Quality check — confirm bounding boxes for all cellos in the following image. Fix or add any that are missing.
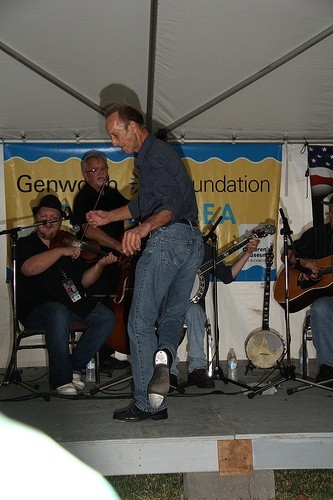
[106,260,133,356]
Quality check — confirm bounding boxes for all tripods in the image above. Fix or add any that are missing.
[167,207,333,400]
[0,214,74,401]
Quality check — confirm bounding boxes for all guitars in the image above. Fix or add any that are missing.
[244,246,285,369]
[273,255,333,313]
[189,225,276,303]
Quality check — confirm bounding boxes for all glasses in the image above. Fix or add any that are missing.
[86,167,108,172]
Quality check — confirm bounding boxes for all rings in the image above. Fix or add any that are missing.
[121,249,127,253]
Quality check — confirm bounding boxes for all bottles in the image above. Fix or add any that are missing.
[228,348,238,383]
[85,358,96,383]
[300,345,309,375]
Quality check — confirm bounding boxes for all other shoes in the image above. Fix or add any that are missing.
[187,368,215,388]
[101,356,129,370]
[56,374,85,396]
[113,405,168,423]
[147,348,174,409]
[316,364,333,388]
[168,373,178,393]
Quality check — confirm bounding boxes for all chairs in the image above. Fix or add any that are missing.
[302,311,313,380]
[3,247,101,385]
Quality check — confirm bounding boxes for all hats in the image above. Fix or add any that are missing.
[32,194,67,217]
[321,195,333,205]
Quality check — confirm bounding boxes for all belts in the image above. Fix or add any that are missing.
[177,219,198,227]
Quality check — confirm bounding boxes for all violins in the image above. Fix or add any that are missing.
[50,230,133,269]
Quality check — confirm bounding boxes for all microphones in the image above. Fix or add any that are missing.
[67,206,80,233]
[290,263,312,275]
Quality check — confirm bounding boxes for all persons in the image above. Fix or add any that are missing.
[102,103,204,422]
[169,234,260,393]
[70,150,149,370]
[13,195,117,395]
[281,196,333,382]
